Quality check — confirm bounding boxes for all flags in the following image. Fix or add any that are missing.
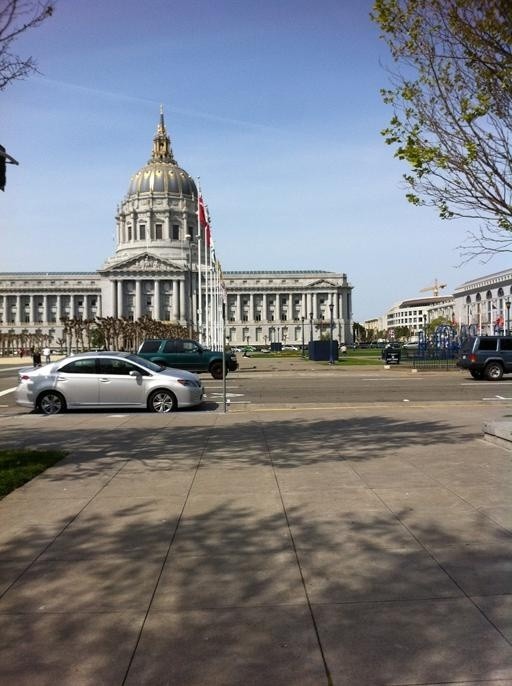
[198,196,226,303]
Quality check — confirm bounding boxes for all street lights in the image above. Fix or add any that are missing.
[301,303,335,355]
[504,302,511,335]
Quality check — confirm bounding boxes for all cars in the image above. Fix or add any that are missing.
[404,342,425,351]
[15,350,208,414]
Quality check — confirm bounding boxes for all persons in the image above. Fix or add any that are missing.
[43,346,52,365]
[20,345,26,357]
[31,345,36,358]
[33,349,42,367]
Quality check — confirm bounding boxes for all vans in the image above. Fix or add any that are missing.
[359,342,398,348]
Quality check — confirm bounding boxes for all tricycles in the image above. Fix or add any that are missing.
[381,348,401,364]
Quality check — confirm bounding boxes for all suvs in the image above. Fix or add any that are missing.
[138,338,239,379]
[456,334,511,380]
